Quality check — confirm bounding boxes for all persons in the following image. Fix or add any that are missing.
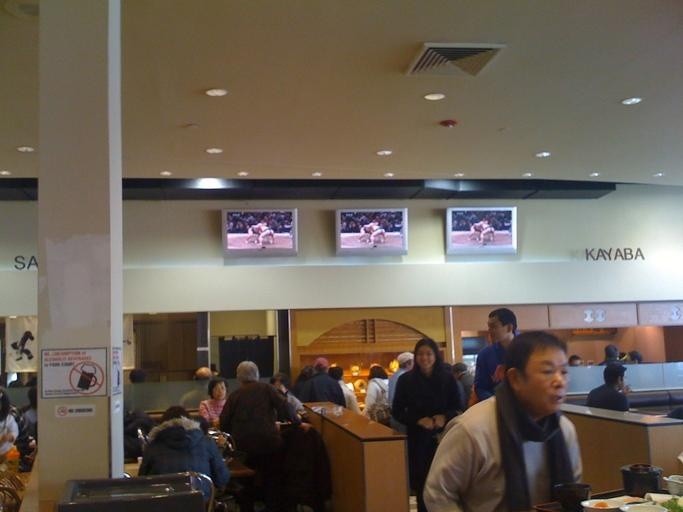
[388,352,416,496]
[452,211,512,245]
[444,363,480,411]
[124,399,157,463]
[392,339,460,512]
[598,345,640,366]
[2,372,37,472]
[423,331,584,512]
[196,361,315,512]
[475,308,517,400]
[227,212,292,249]
[342,212,402,248]
[139,405,230,512]
[304,357,360,413]
[585,363,630,411]
[361,363,393,429]
[129,369,145,383]
[569,355,582,367]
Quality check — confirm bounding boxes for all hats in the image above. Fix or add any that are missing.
[312,358,331,371]
[397,352,414,366]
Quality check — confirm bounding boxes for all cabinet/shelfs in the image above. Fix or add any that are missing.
[343,372,393,409]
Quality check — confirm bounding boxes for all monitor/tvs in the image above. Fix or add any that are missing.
[462,348,481,377]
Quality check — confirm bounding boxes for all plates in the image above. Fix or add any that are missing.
[579,492,667,512]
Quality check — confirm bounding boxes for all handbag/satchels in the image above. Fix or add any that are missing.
[368,379,391,424]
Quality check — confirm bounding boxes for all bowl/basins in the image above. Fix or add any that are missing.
[552,482,590,508]
[663,473,683,495]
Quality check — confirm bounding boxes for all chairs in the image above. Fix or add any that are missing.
[137,428,149,449]
